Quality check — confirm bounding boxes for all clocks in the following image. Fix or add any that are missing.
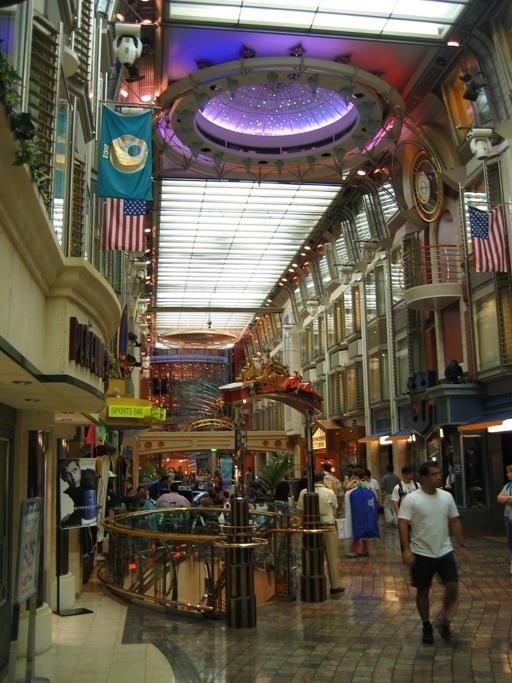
[410,151,443,223]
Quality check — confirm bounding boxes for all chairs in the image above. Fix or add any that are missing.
[114,495,232,548]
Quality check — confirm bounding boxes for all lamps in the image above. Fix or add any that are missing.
[149,44,407,185]
[160,330,237,351]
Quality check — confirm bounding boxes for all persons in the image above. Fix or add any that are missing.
[296,472,347,593]
[319,461,418,560]
[397,460,465,643]
[497,465,512,574]
[60,459,96,527]
[117,466,274,509]
[443,462,455,488]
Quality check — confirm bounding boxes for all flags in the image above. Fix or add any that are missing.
[101,196,146,253]
[95,100,156,200]
[468,202,509,273]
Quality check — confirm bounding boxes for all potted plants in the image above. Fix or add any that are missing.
[250,448,298,560]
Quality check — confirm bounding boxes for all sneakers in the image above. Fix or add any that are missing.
[421,620,454,645]
[328,586,347,598]
[343,549,370,559]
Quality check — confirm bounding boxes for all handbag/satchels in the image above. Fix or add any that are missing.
[335,518,346,541]
